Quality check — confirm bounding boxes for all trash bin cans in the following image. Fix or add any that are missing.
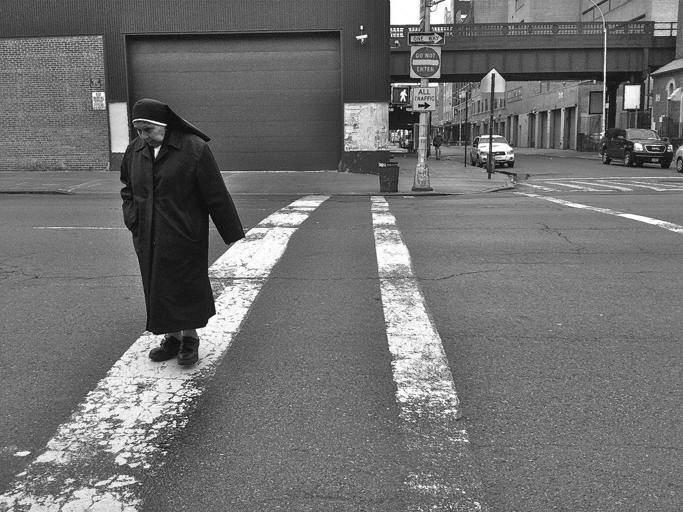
[377,160,399,192]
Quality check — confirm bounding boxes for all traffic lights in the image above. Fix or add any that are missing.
[392,86,410,105]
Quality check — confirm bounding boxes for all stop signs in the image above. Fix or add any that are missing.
[411,47,440,79]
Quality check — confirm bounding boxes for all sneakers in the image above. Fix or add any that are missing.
[149,335,181,361]
[177,335,200,366]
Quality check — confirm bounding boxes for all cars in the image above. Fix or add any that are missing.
[676,144,682,173]
[390,132,411,147]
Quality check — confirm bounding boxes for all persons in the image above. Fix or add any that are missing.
[118,97,246,368]
[432,131,444,160]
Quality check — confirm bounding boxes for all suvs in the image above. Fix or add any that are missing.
[598,128,673,170]
[470,133,515,169]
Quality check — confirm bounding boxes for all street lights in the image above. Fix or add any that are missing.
[448,95,462,148]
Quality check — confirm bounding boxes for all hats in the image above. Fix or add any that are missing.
[132,98,169,126]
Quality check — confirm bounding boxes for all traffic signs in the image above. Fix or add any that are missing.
[408,32,444,46]
[411,87,437,113]
